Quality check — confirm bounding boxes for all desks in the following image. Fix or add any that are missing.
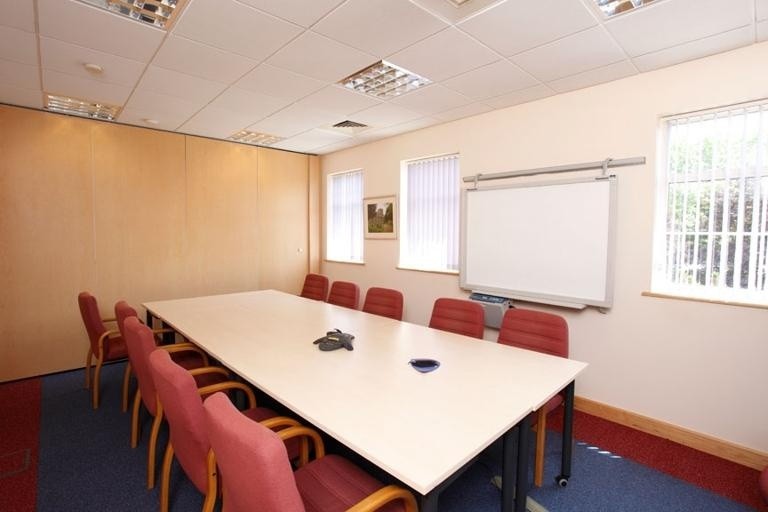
[139,290,593,512]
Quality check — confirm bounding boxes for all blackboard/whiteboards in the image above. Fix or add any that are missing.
[460,172,620,310]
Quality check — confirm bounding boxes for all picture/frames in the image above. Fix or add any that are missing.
[362,194,400,241]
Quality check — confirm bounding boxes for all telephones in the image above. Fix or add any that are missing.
[313,328,355,350]
